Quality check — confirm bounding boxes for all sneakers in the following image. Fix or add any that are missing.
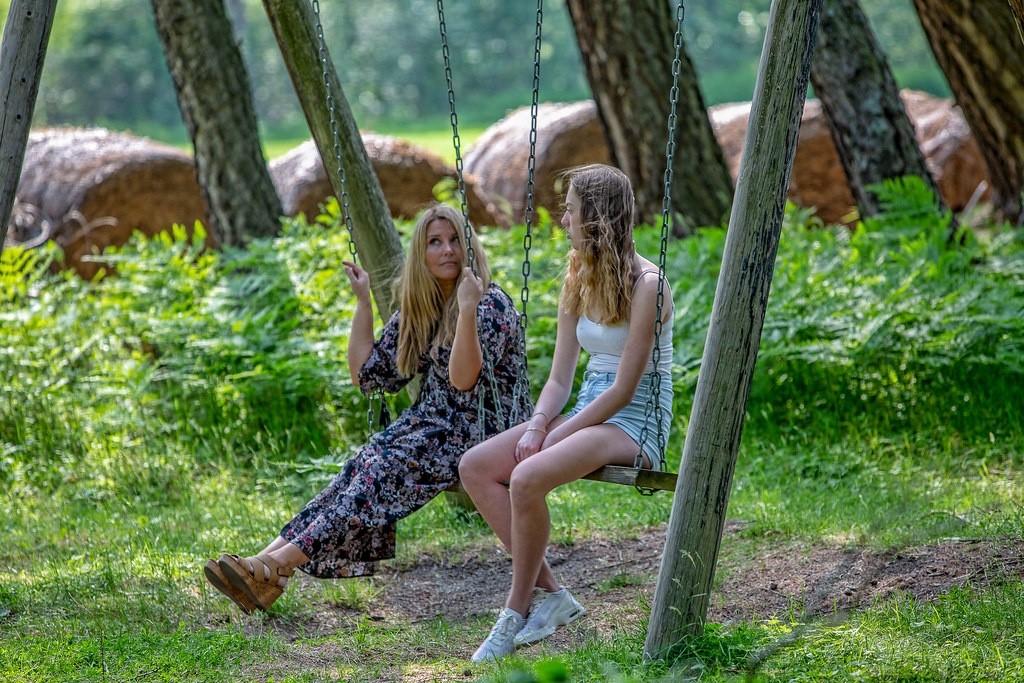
[470,607,528,666]
[513,585,586,651]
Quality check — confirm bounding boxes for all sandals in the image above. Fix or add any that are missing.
[218,554,295,612]
[204,559,256,616]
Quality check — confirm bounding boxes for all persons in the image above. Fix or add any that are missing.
[458,162,675,664]
[204,206,533,621]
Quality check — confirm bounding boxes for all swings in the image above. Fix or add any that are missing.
[506,0,688,496]
[310,0,477,494]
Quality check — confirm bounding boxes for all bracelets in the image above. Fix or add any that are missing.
[526,411,550,434]
